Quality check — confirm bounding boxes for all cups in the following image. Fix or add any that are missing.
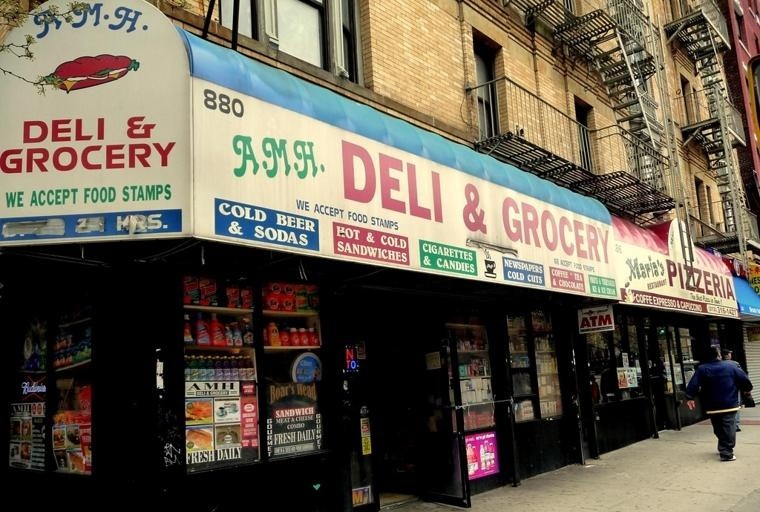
[231,404,236,412]
[219,407,225,416]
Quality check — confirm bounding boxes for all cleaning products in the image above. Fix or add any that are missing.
[242,317,255,347]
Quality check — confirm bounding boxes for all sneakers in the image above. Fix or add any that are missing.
[736,429,741,431]
[721,456,736,460]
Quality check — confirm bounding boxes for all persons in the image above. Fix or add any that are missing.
[685,344,755,461]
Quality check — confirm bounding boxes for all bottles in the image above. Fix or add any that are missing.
[184,355,255,382]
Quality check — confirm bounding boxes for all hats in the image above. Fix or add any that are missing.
[722,348,734,353]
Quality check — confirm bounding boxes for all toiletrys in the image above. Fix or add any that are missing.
[194,312,213,346]
[229,321,245,347]
[224,322,235,347]
[208,312,228,346]
[183,314,198,344]
